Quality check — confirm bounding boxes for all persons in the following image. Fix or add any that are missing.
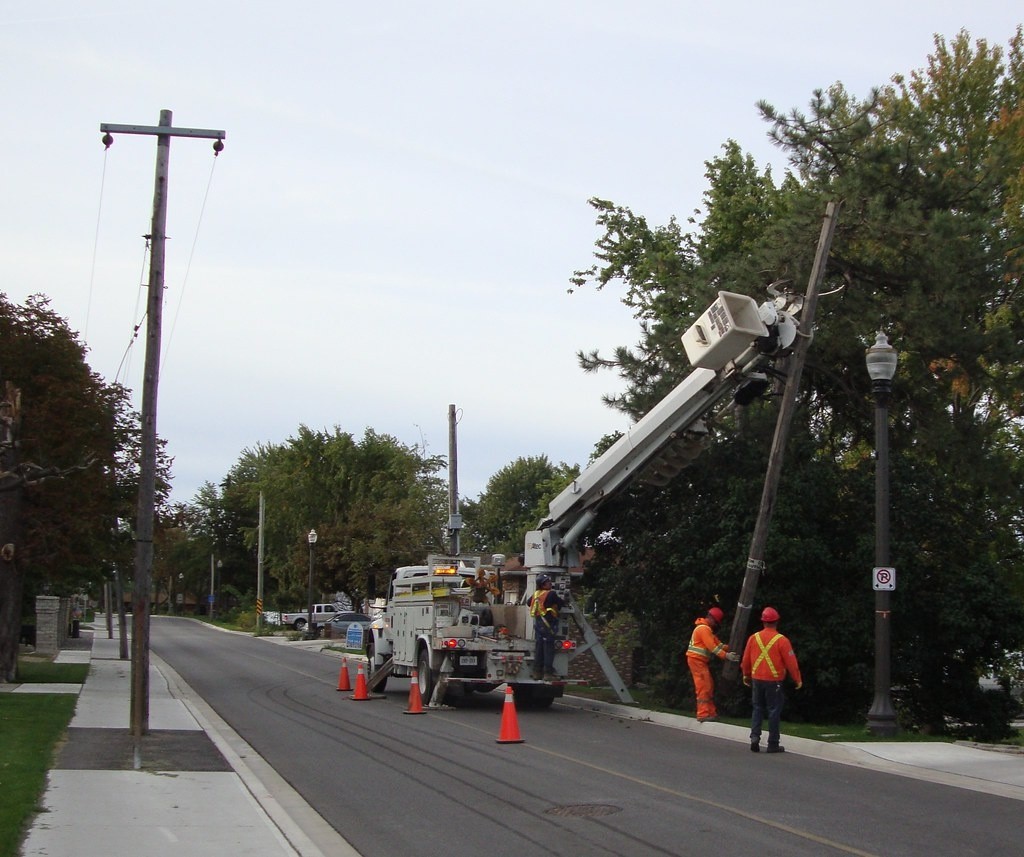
[686,608,741,722]
[742,608,802,753]
[527,573,562,681]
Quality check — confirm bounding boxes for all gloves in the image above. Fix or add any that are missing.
[743,676,752,689]
[795,682,803,690]
[725,652,741,662]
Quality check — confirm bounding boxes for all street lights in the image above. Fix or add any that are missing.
[864,333,899,735]
[178,573,184,616]
[217,560,223,615]
[308,529,317,630]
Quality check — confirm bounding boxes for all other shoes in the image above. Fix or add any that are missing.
[697,716,719,722]
[532,672,545,680]
[750,743,760,752]
[767,746,784,753]
[543,674,562,681]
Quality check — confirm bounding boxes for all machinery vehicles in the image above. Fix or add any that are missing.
[365,278,845,709]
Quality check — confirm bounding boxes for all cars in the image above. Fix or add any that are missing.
[303,613,374,632]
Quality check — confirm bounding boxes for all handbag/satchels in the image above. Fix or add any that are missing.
[775,677,795,699]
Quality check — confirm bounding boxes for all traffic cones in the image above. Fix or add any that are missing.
[402,671,428,715]
[349,664,372,701]
[335,657,354,691]
[496,686,527,744]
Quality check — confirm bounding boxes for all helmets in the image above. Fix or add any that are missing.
[535,574,549,590]
[709,607,724,624]
[760,606,781,622]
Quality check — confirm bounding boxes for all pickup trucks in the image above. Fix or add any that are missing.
[277,604,344,630]
